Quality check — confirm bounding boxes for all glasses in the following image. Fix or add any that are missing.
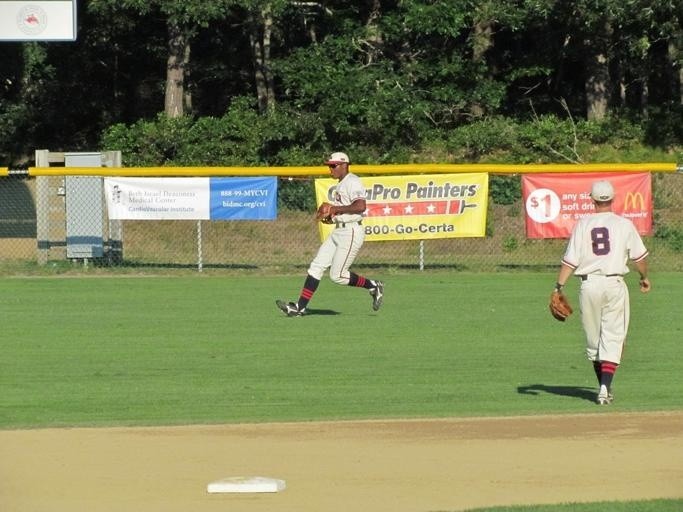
[329,164,338,168]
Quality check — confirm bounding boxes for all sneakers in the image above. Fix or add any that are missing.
[369,280,384,310]
[598,391,614,406]
[276,300,306,317]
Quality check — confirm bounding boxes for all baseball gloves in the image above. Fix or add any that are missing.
[315,203,335,223]
[551,289,573,322]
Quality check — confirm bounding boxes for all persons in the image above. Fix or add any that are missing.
[275,151,383,317]
[548,180,651,404]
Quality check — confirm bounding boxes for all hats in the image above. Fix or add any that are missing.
[324,152,349,164]
[591,181,614,202]
